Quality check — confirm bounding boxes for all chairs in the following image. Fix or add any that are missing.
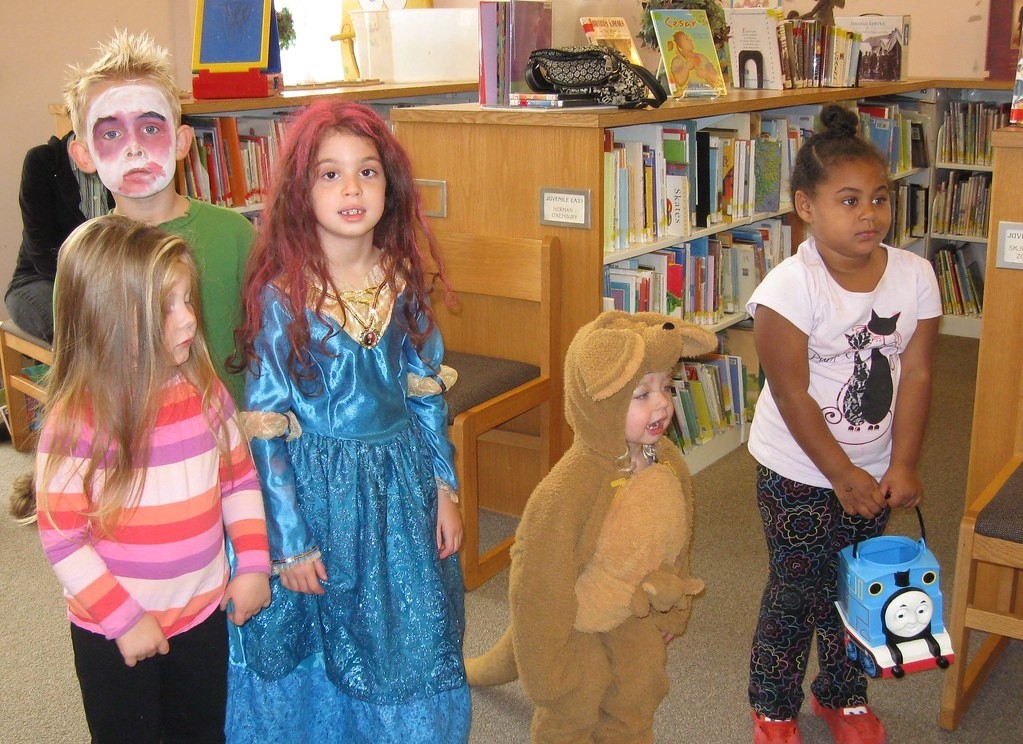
[0,319,53,452]
[409,229,562,590]
[939,402,1023,733]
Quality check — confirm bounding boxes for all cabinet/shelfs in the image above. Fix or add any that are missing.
[387,80,1023,618]
[48,80,481,226]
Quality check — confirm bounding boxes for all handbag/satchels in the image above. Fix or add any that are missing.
[525,47,669,110]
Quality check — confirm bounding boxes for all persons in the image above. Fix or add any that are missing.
[466,309,720,744]
[35,213,274,743]
[231,102,473,743]
[4,26,265,526]
[746,104,942,744]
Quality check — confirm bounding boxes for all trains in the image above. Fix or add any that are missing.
[834,535,956,679]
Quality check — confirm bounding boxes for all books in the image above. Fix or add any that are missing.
[179,93,464,237]
[510,8,1023,462]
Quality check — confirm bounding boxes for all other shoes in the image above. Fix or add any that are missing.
[753,706,800,743]
[811,689,885,744]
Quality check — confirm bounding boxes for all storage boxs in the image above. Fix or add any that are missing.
[835,13,912,82]
